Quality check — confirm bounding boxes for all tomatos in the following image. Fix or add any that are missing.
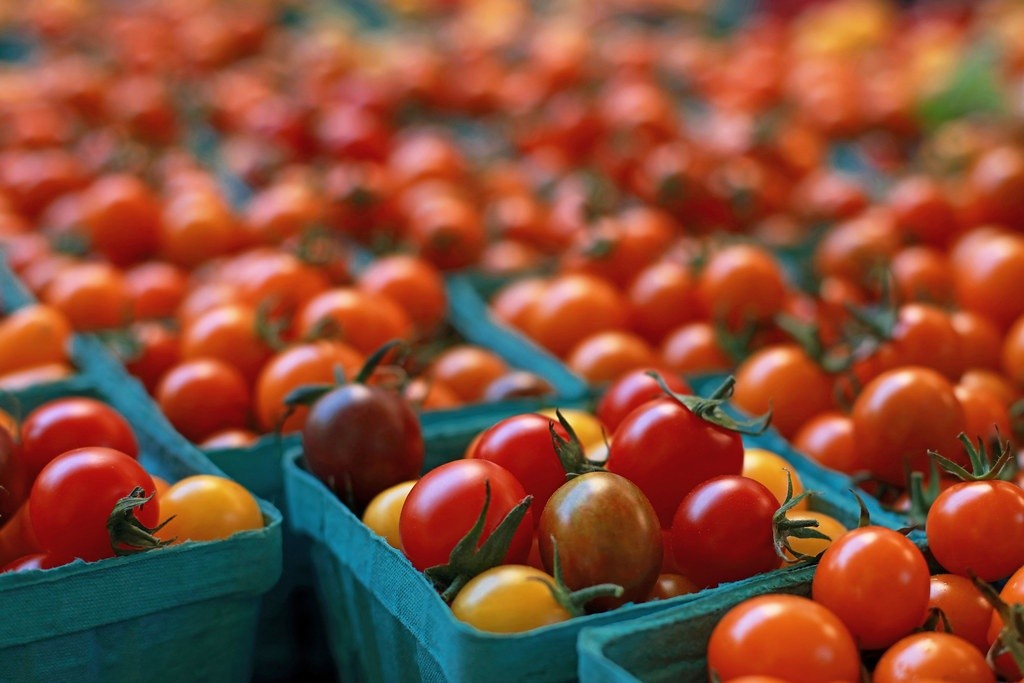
[0,0,1024,683]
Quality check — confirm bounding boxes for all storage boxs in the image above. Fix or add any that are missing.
[0,0,1024,683]
